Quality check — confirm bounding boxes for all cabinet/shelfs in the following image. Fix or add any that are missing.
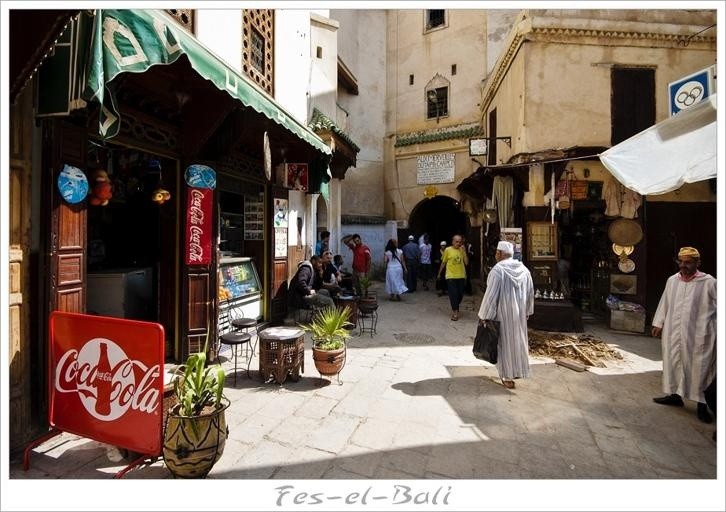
[217,253,265,340]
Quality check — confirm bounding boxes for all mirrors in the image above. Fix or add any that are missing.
[524,219,559,263]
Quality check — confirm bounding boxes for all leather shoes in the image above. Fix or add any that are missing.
[653,396,683,407]
[697,405,712,423]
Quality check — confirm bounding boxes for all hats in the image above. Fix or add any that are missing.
[678,247,701,258]
[408,235,415,241]
[496,241,514,251]
[440,241,447,246]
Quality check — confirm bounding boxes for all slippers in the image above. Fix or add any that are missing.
[451,314,458,321]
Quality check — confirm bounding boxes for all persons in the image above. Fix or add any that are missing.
[344,233,371,298]
[478,240,535,390]
[650,246,717,424]
[290,230,355,314]
[383,231,475,321]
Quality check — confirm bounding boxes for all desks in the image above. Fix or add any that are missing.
[528,297,586,333]
[258,325,306,385]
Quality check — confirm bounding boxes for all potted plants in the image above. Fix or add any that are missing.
[299,303,355,379]
[163,336,234,478]
[350,270,378,315]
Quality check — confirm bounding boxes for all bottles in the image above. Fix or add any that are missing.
[93,342,111,416]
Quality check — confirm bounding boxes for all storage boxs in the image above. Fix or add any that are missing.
[606,307,647,335]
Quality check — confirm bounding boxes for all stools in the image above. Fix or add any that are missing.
[215,330,256,389]
[231,316,259,359]
[292,300,316,325]
